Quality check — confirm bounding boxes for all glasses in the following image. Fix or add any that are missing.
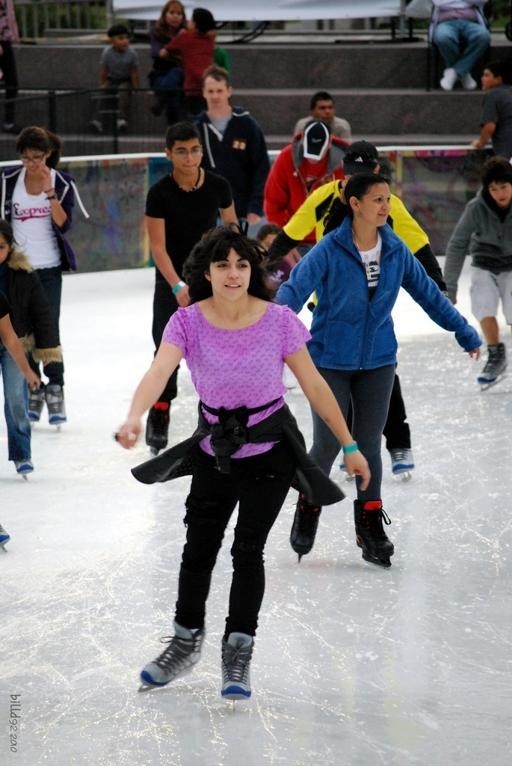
[21,151,46,163]
[173,147,202,156]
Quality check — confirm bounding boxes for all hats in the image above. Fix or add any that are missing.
[304,120,329,161]
[343,141,380,176]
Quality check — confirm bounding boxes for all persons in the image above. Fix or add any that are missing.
[0,0,512,703]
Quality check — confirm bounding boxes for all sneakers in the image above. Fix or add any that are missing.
[45,384,66,424]
[441,67,458,92]
[89,121,103,132]
[3,124,21,135]
[291,493,322,554]
[340,462,346,472]
[390,449,414,474]
[14,462,33,473]
[147,404,169,448]
[354,499,393,558]
[459,73,478,90]
[222,634,254,700]
[27,383,44,422]
[117,120,128,132]
[0,526,10,546]
[478,345,505,383]
[141,620,203,687]
